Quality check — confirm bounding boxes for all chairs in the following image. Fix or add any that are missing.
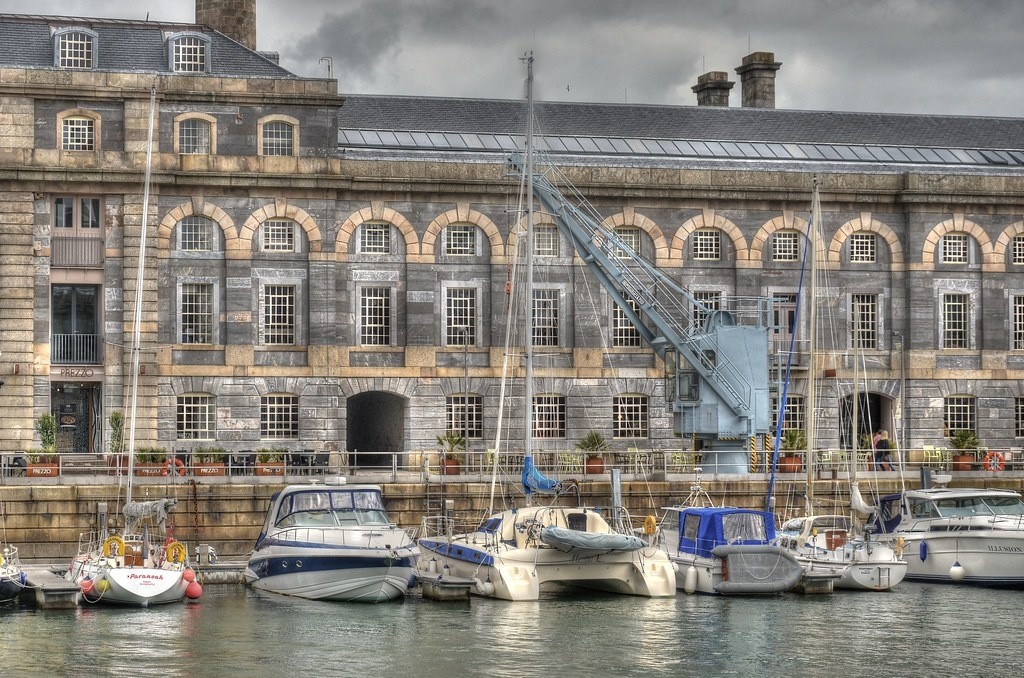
[614,447,697,474]
[175,448,190,472]
[222,450,257,476]
[1012,451,1024,472]
[0,452,27,477]
[286,448,330,476]
[473,448,583,474]
[923,445,950,468]
[819,451,869,471]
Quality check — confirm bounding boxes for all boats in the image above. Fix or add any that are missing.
[242,473,422,603]
[1,544,27,597]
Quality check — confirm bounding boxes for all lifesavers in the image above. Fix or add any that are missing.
[644,516,656,535]
[20,572,26,585]
[161,458,185,477]
[103,536,125,557]
[983,452,1005,471]
[166,542,185,562]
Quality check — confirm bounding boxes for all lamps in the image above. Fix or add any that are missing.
[14,364,19,375]
[825,369,836,378]
[140,365,145,374]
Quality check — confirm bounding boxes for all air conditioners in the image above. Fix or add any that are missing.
[970,446,989,465]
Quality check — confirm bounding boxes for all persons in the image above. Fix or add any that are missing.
[871,428,896,471]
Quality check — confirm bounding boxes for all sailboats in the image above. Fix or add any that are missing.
[420,52,1024,606]
[68,84,201,609]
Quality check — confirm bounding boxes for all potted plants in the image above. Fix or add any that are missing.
[134,447,168,476]
[192,446,226,477]
[573,431,612,474]
[106,411,129,475]
[436,431,467,475]
[25,413,59,477]
[779,429,807,473]
[949,430,979,470]
[256,448,286,476]
